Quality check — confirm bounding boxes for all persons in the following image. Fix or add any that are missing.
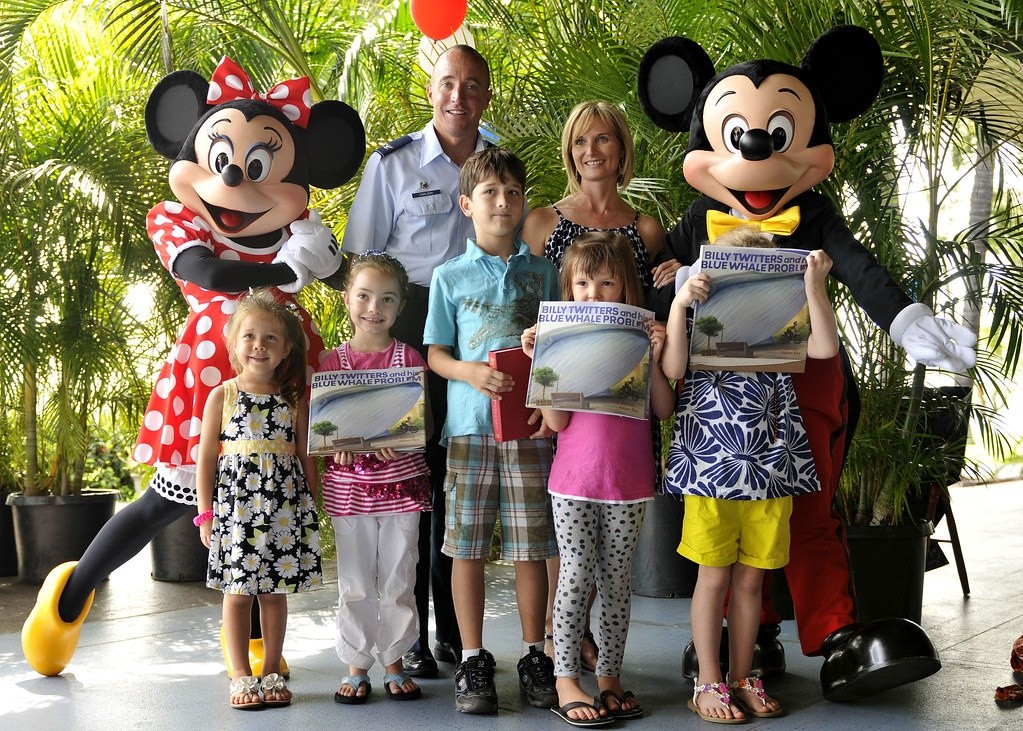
[197,284,317,709]
[341,45,502,679]
[520,99,683,676]
[520,230,676,727]
[660,229,840,723]
[422,147,563,713]
[320,251,430,705]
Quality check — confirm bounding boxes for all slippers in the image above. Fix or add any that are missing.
[230,675,262,710]
[383,671,421,700]
[261,672,291,705]
[599,685,644,717]
[335,674,371,704]
[551,692,615,726]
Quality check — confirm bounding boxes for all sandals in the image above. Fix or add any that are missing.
[686,673,748,723]
[725,671,784,716]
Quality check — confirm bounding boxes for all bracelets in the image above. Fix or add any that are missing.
[193,510,213,527]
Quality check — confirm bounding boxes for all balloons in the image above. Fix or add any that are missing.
[410,0,467,41]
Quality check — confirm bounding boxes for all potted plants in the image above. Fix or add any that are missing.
[0,0,1023,623]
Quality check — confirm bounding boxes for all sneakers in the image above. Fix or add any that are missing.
[455,649,499,715]
[517,644,560,708]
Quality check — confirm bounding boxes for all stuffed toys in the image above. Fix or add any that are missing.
[20,54,365,676]
[635,23,979,701]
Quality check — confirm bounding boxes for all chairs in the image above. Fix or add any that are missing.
[871,387,969,596]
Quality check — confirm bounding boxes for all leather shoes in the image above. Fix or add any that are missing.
[403,650,439,674]
[435,640,495,668]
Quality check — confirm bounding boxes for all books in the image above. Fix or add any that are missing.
[488,346,543,443]
[690,245,815,374]
[524,300,656,421]
[306,366,427,456]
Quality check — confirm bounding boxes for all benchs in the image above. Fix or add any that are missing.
[715,341,753,358]
[332,436,370,451]
[551,393,590,409]
